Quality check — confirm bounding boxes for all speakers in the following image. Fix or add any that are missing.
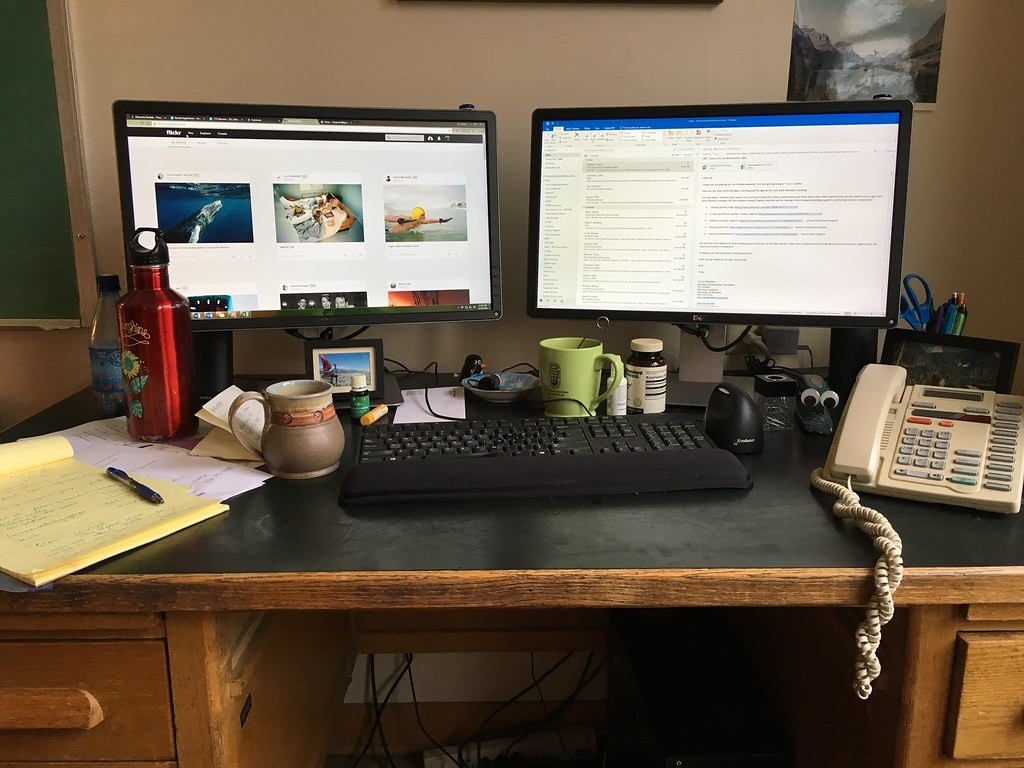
[189,331,233,410]
[829,327,877,413]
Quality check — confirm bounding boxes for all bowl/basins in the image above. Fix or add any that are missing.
[462,372,540,403]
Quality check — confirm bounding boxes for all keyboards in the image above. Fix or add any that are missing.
[338,412,754,504]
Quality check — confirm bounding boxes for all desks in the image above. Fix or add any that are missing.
[0,371,1023,767]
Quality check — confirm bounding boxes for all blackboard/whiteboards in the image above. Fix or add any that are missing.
[0,0,101,331]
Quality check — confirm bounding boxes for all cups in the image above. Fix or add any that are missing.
[538,337,623,419]
[228,379,345,479]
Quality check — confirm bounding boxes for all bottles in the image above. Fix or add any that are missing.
[87,273,128,417]
[607,354,627,416]
[626,338,666,414]
[115,227,199,442]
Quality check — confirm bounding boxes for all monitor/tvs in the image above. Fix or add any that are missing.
[111,99,504,408]
[528,98,913,406]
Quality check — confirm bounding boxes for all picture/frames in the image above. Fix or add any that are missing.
[303,339,383,400]
[879,327,1020,395]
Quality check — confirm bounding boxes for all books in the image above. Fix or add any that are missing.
[0,436,228,589]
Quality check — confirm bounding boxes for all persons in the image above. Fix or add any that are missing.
[296,293,354,310]
[385,207,453,234]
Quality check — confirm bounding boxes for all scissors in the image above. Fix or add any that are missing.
[899,273,932,331]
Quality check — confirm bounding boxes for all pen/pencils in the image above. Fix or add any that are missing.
[936,291,969,336]
[105,465,166,505]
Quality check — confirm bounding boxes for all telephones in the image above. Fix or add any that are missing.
[821,364,1024,514]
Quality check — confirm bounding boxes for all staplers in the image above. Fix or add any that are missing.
[796,372,839,435]
